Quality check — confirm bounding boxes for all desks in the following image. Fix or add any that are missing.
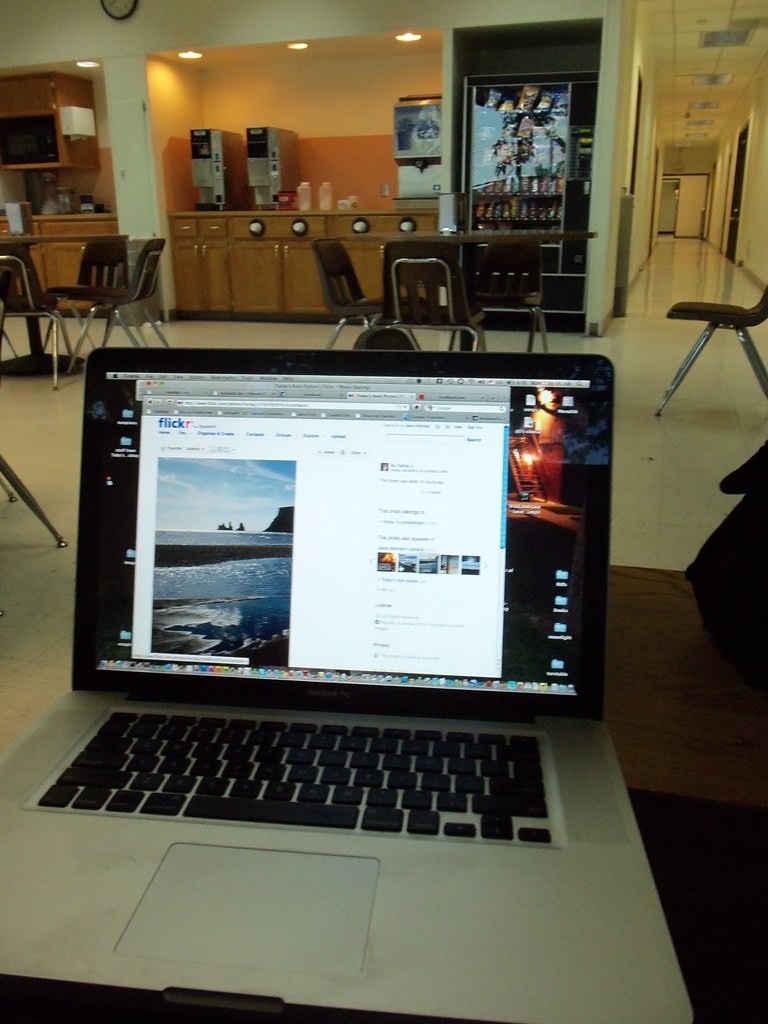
[0,230,129,375]
[338,228,598,351]
[607,563,768,815]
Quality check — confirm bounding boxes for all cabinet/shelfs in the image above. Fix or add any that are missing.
[0,215,118,318]
[335,215,436,302]
[230,217,331,315]
[0,71,101,171]
[168,218,232,312]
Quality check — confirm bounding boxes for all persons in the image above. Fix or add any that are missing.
[222,523,232,529]
[237,523,244,530]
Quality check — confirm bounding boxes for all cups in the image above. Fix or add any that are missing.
[338,200,348,210]
[348,196,359,210]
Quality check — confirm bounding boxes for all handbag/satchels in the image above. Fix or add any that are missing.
[685,440,768,692]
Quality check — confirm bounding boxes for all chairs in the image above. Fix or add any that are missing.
[654,283,768,417]
[0,236,168,389]
[450,242,550,353]
[380,237,485,352]
[311,237,392,349]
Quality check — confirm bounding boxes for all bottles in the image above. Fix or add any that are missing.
[297,182,310,210]
[57,187,73,214]
[319,182,331,211]
[477,175,563,218]
[40,177,58,215]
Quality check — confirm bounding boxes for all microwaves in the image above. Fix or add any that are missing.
[1,114,59,165]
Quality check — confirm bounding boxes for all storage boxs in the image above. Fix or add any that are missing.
[278,190,298,210]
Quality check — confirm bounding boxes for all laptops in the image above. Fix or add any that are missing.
[0,347,694,1023]
